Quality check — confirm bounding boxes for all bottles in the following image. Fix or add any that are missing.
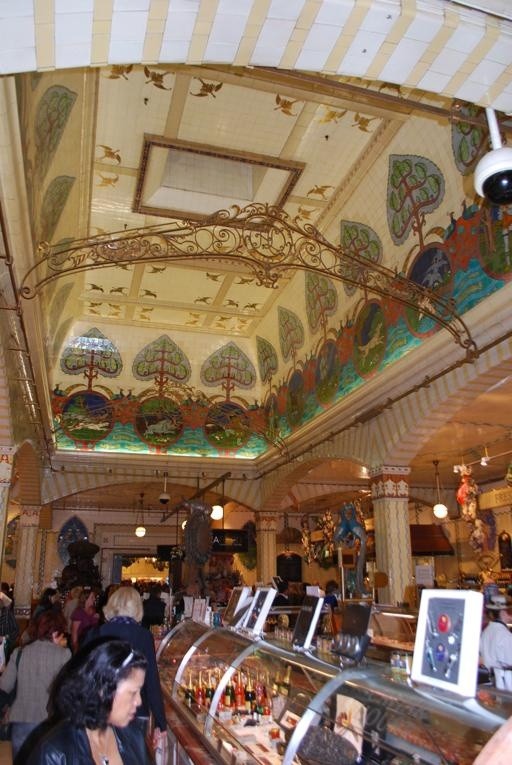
[273,663,294,696]
[181,660,271,726]
[335,633,361,656]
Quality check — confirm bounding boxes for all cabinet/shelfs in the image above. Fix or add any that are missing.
[156,619,511,765]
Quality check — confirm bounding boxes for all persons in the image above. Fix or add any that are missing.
[271,582,289,605]
[479,596,512,671]
[0,579,235,765]
[323,581,338,607]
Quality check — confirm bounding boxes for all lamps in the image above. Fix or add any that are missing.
[428,459,449,520]
[452,454,489,474]
[158,475,225,530]
[132,493,147,537]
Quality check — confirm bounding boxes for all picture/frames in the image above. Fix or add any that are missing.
[243,586,275,636]
[410,586,486,701]
[221,585,250,629]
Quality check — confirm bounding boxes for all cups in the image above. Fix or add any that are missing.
[212,613,224,627]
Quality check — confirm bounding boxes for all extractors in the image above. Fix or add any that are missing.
[316,525,454,557]
